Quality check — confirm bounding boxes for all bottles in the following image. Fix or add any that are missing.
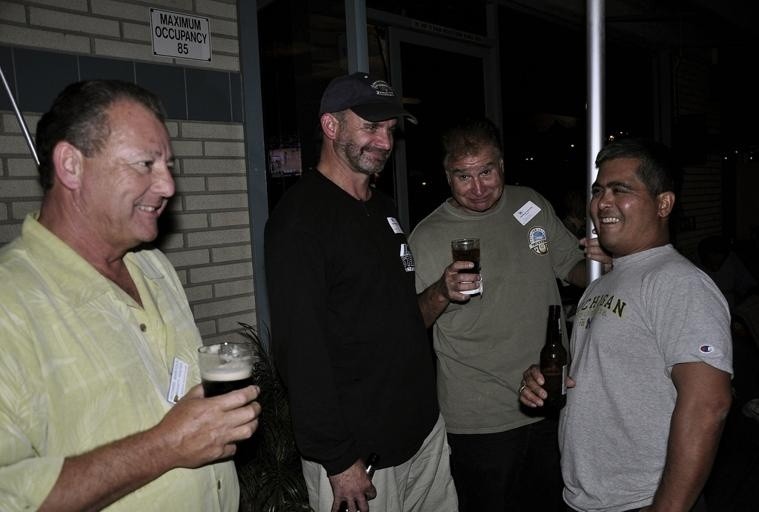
[539,301,567,412]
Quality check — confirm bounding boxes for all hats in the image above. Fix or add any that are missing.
[319,72,419,125]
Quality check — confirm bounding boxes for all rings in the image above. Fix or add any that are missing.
[518,385,527,394]
[263,70,461,512]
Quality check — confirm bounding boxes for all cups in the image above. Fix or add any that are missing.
[197,343,254,399]
[450,237,485,297]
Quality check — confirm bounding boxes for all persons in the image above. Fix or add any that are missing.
[407,131,616,512]
[0,75,265,512]
[517,139,739,512]
